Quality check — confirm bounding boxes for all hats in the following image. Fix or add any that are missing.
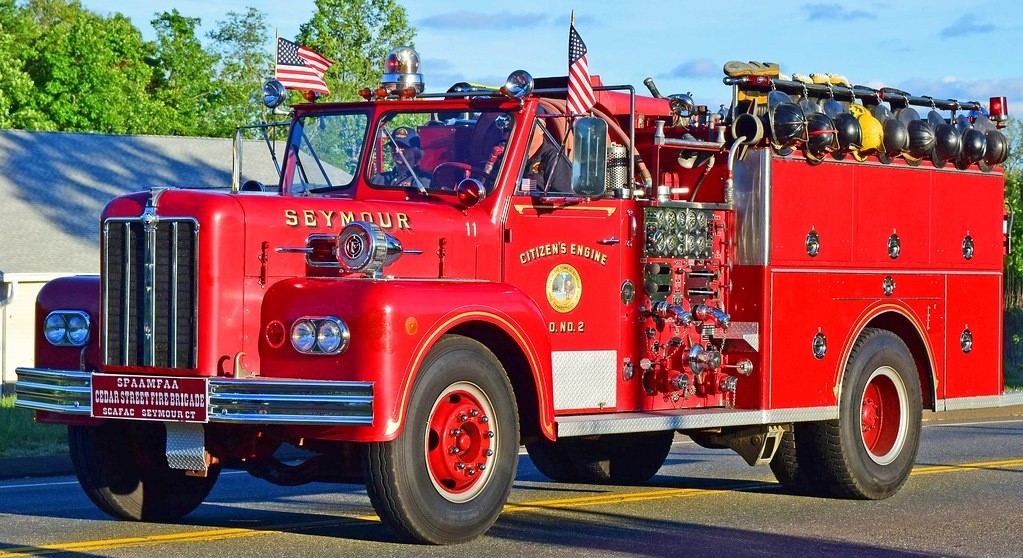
[384,126,420,149]
[535,104,546,120]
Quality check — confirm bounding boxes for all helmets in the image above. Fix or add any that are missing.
[766,91,1009,172]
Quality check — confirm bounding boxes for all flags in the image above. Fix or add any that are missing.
[562,23,595,118]
[275,36,337,95]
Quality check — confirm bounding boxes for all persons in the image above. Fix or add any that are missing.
[485,104,576,199]
[373,127,433,191]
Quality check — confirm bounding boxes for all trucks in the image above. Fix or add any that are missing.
[15,8,1010,546]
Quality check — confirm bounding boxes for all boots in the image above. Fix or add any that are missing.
[723,60,779,123]
[793,73,912,116]
